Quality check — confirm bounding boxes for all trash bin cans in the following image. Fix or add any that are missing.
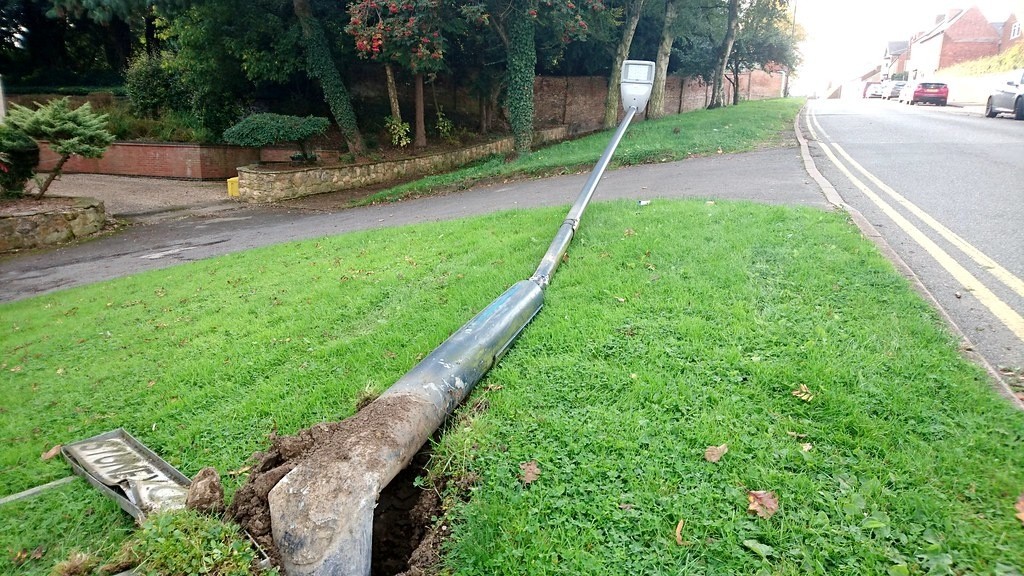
[227,177,240,197]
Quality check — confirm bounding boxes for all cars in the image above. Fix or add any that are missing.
[985,70,1024,120]
[862,81,949,106]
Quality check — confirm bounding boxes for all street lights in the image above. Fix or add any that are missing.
[269,60,656,576]
